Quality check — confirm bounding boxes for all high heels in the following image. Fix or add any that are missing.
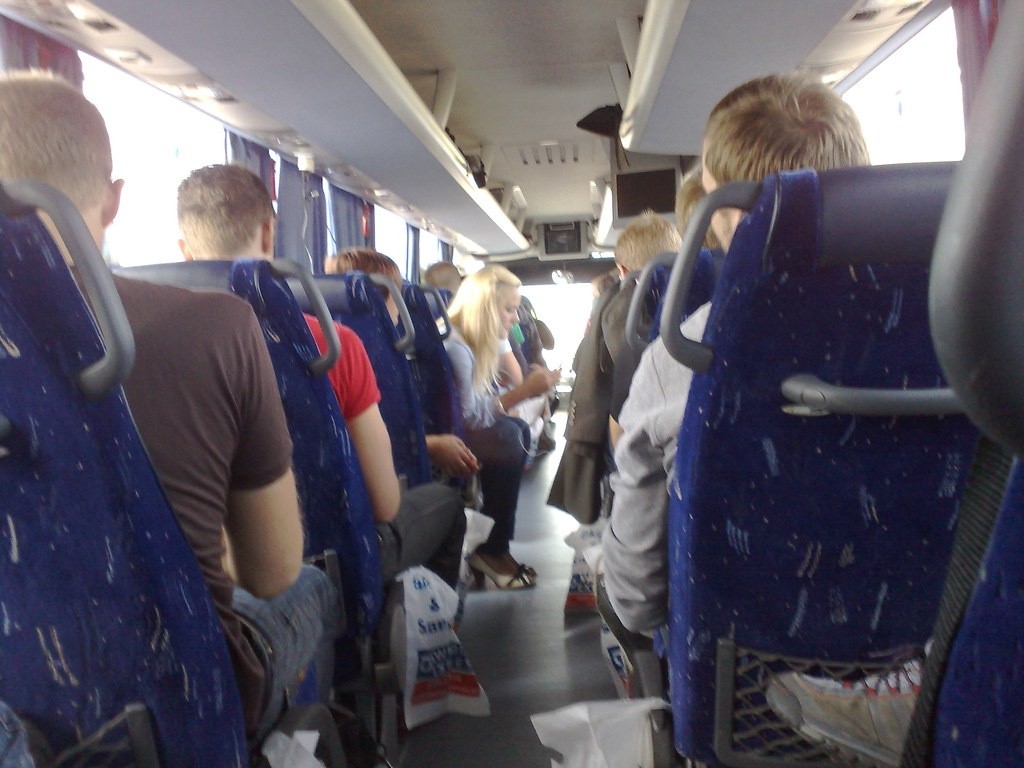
[467,545,538,591]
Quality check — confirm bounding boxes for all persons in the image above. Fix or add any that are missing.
[430,262,564,590]
[172,163,470,737]
[314,242,566,485]
[760,630,947,767]
[585,67,871,702]
[1,69,353,768]
[546,158,726,600]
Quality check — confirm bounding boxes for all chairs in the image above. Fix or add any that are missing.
[276,272,434,490]
[899,0,1024,768]
[100,257,399,768]
[397,280,465,491]
[507,296,548,380]
[595,248,727,706]
[663,158,973,768]
[0,178,349,768]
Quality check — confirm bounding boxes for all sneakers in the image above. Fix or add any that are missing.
[759,646,926,768]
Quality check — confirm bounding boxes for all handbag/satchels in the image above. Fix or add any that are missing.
[563,517,604,614]
[529,696,672,768]
[394,564,491,731]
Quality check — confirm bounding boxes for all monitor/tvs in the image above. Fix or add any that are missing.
[538,221,589,262]
[612,163,683,230]
